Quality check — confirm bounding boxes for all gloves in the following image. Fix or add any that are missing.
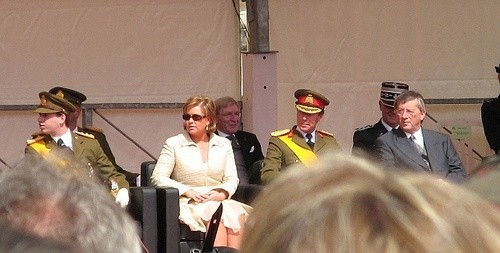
[115,188,129,207]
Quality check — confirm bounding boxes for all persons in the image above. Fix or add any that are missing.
[213,64,500,253]
[0,86,142,253]
[149,96,255,249]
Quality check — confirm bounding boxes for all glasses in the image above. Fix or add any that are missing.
[217,113,241,118]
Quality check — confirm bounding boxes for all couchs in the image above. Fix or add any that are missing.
[129,187,157,253]
[140,161,264,253]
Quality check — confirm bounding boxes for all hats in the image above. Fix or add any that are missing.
[183,114,207,121]
[33,91,77,116]
[294,88,330,113]
[49,86,87,106]
[380,81,409,107]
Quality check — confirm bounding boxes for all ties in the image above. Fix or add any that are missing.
[306,133,315,152]
[226,135,241,150]
[409,135,429,166]
[57,138,63,146]
